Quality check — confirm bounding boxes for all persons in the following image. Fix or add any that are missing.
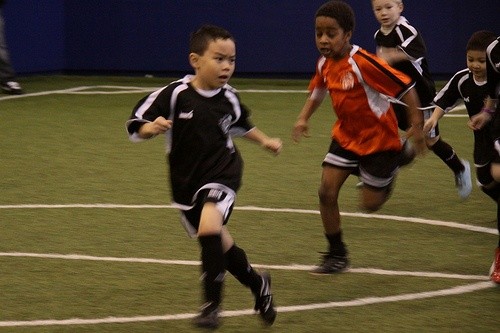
[422,29,500,204]
[124,24,282,330]
[368,0,473,200]
[292,0,426,275]
[468,32,500,286]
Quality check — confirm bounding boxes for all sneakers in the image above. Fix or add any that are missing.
[453,160,472,198]
[0,81,22,94]
[194,302,218,329]
[309,250,349,276]
[492,247,500,284]
[255,271,277,325]
